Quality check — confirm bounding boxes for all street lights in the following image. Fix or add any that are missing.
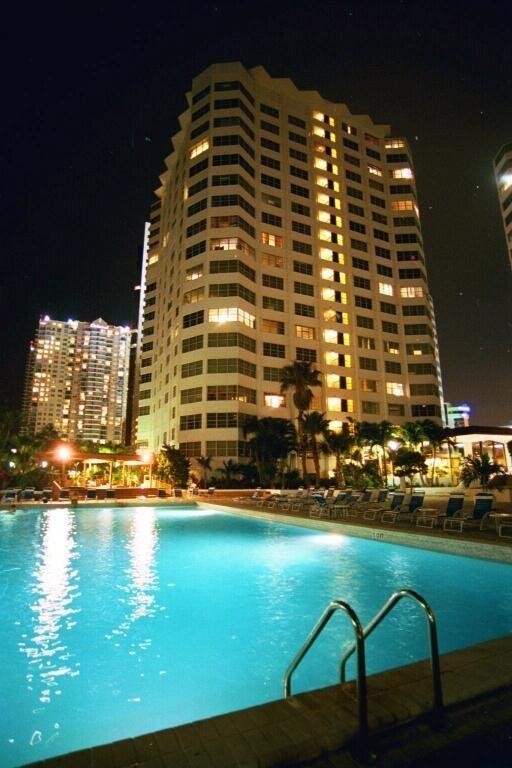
[59,448,69,473]
[143,453,153,488]
[8,447,17,479]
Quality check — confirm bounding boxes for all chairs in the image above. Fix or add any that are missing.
[253,474,508,542]
[1,485,193,505]
